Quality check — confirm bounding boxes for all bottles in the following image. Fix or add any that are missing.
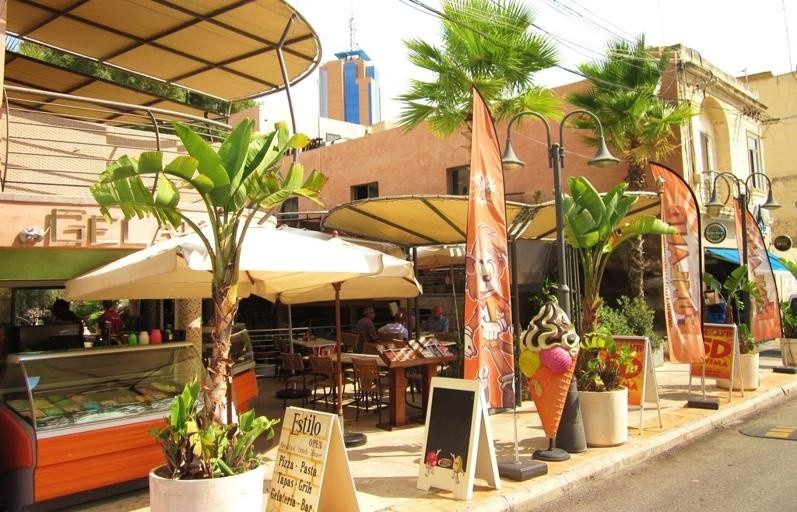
[128,323,174,345]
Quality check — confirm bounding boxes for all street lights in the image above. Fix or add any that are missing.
[501,109,621,453]
[704,171,781,352]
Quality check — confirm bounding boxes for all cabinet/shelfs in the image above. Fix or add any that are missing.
[1,342,213,512]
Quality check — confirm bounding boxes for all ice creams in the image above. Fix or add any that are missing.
[516,300,581,440]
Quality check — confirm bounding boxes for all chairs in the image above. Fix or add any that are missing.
[271,327,411,424]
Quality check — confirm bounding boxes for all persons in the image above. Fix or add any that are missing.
[375,311,408,341]
[423,304,449,336]
[397,307,416,332]
[96,300,124,342]
[49,299,84,349]
[355,307,379,342]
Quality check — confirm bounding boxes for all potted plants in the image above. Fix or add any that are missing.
[700,263,767,390]
[551,174,678,447]
[87,113,328,512]
[778,294,797,372]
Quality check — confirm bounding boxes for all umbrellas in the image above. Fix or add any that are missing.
[59,210,424,432]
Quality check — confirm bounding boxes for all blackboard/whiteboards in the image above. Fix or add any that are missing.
[418,376,482,492]
